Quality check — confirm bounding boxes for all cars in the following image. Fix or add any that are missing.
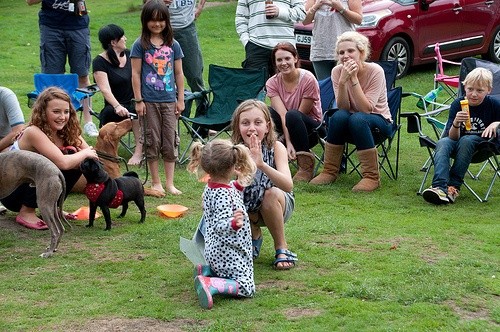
[292,0,499,81]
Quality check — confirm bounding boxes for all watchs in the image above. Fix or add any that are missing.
[339,7,347,15]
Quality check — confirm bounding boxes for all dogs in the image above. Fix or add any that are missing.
[72,118,133,191]
[79,156,147,232]
[0,149,72,258]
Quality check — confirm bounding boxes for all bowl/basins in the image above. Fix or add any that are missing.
[157,204,189,217]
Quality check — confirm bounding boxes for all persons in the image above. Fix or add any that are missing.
[421,67,500,206]
[310,31,396,192]
[0,86,99,229]
[304,0,362,83]
[187,138,257,309]
[163,0,217,136]
[91,23,144,165]
[265,41,324,182]
[129,0,183,196]
[235,0,306,77]
[231,99,297,271]
[25,0,99,137]
[0,86,25,151]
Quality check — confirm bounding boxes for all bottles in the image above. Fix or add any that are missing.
[265,0,274,19]
[461,100,471,130]
[418,85,442,110]
[77,0,87,16]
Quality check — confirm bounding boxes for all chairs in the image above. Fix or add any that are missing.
[27,44,500,202]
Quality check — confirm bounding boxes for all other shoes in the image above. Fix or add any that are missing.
[0,206,7,212]
[64,213,77,220]
[16,215,48,230]
[209,129,217,136]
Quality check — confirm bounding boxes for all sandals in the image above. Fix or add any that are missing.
[252,231,263,259]
[274,248,298,270]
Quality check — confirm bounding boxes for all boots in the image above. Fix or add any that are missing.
[193,264,218,277]
[352,148,381,192]
[194,275,239,309]
[310,141,345,185]
[293,151,315,182]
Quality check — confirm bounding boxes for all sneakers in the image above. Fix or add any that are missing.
[84,121,99,137]
[422,187,449,205]
[447,186,459,204]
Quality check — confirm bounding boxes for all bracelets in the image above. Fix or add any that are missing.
[352,82,359,86]
[114,104,120,109]
[309,6,316,14]
[452,122,460,129]
[131,98,144,102]
